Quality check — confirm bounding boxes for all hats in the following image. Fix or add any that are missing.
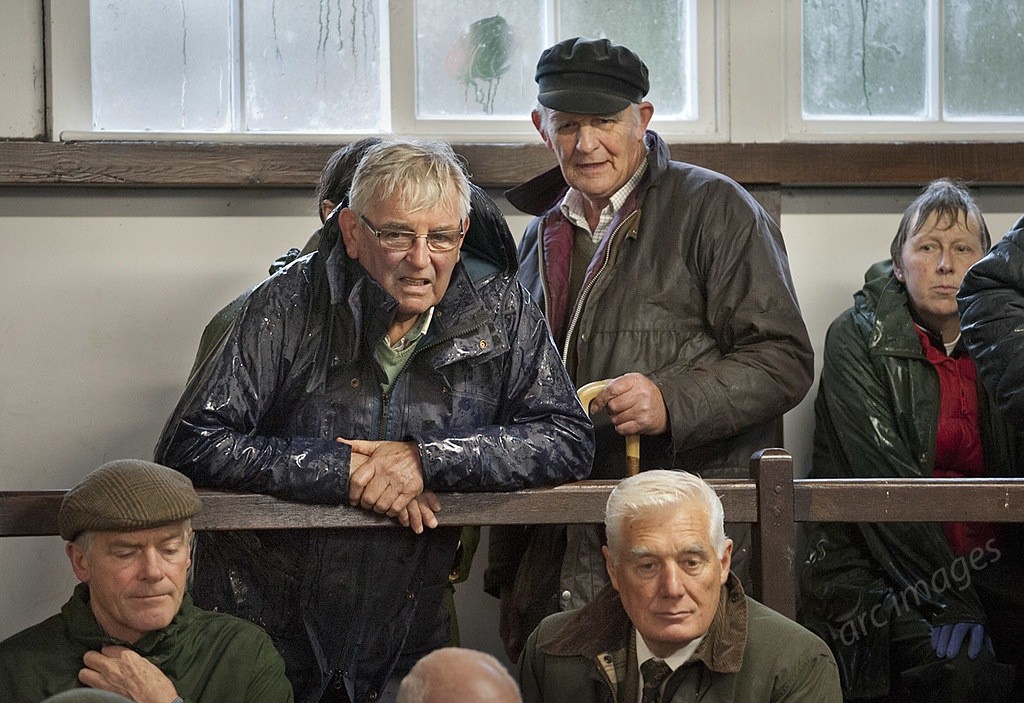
[534,37,649,114]
[58,457,201,542]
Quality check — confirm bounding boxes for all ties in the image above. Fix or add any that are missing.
[641,658,672,703]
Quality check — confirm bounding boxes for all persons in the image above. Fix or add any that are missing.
[1,136,1024,703]
[483,35,815,664]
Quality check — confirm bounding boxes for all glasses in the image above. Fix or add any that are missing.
[356,212,463,252]
